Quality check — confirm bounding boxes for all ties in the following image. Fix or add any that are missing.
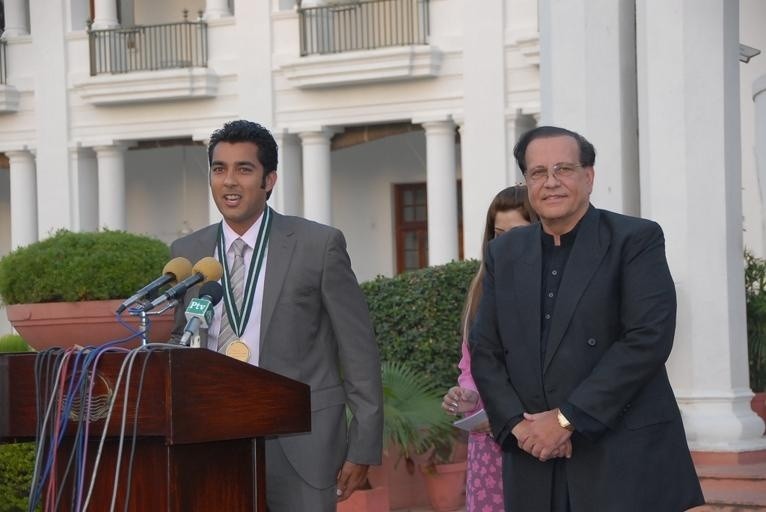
[216,237,247,355]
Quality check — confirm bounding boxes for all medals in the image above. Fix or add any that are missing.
[220,339,251,363]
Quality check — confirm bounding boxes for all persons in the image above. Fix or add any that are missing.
[466,125,706,512]
[441,183,540,512]
[162,120,384,512]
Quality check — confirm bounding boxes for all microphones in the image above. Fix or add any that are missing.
[179,280,223,346]
[143,256,224,312]
[116,257,193,314]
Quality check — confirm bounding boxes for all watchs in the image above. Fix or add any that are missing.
[556,408,575,431]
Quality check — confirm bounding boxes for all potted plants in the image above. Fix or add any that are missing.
[0,228,176,350]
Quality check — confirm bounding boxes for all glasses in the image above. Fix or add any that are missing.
[529,161,584,178]
